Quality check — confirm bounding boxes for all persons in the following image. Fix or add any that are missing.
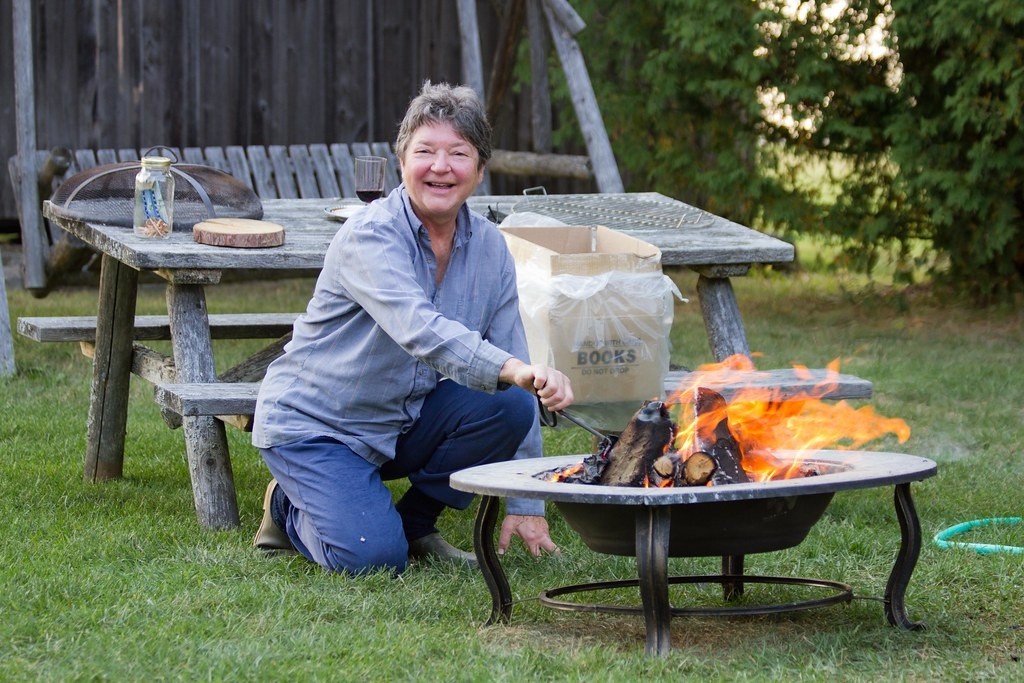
[249,77,575,584]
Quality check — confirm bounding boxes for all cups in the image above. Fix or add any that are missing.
[355,155,387,204]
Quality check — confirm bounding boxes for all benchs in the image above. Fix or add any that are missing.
[14,311,306,345]
[155,366,874,417]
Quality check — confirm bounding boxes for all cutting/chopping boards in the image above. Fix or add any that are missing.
[192,218,286,249]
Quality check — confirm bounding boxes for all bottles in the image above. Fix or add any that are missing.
[132,154,175,238]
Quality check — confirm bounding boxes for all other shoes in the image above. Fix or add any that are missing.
[252,478,299,559]
[408,532,480,568]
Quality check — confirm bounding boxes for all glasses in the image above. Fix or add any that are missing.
[486,205,508,224]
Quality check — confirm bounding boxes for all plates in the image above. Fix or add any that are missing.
[323,203,365,222]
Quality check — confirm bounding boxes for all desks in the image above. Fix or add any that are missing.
[41,193,795,534]
